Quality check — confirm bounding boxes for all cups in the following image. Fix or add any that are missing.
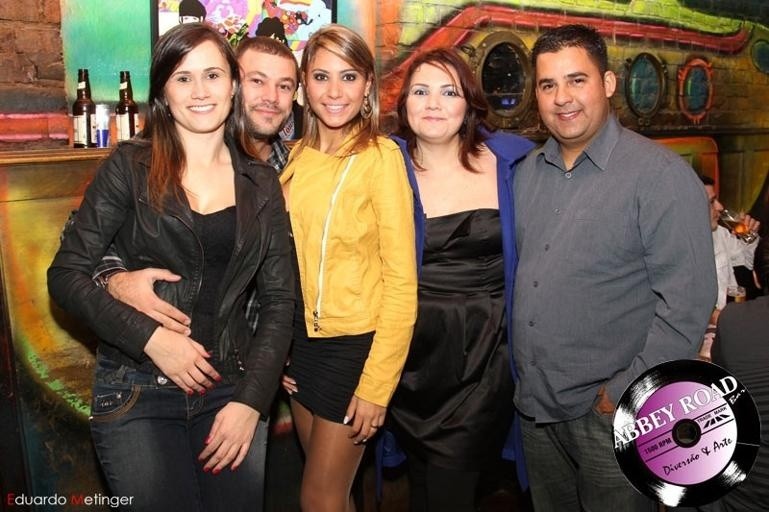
[719,209,755,244]
[97,105,110,148]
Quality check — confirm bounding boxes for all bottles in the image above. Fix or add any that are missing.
[72,68,98,148]
[115,71,140,146]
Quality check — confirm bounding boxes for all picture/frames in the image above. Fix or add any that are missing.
[149,0,338,107]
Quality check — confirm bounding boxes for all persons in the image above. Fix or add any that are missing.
[83,33,308,512]
[508,26,719,511]
[700,176,764,325]
[350,47,543,510]
[278,22,417,511]
[47,20,298,511]
[706,238,768,511]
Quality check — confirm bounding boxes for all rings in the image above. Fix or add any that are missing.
[369,424,379,429]
